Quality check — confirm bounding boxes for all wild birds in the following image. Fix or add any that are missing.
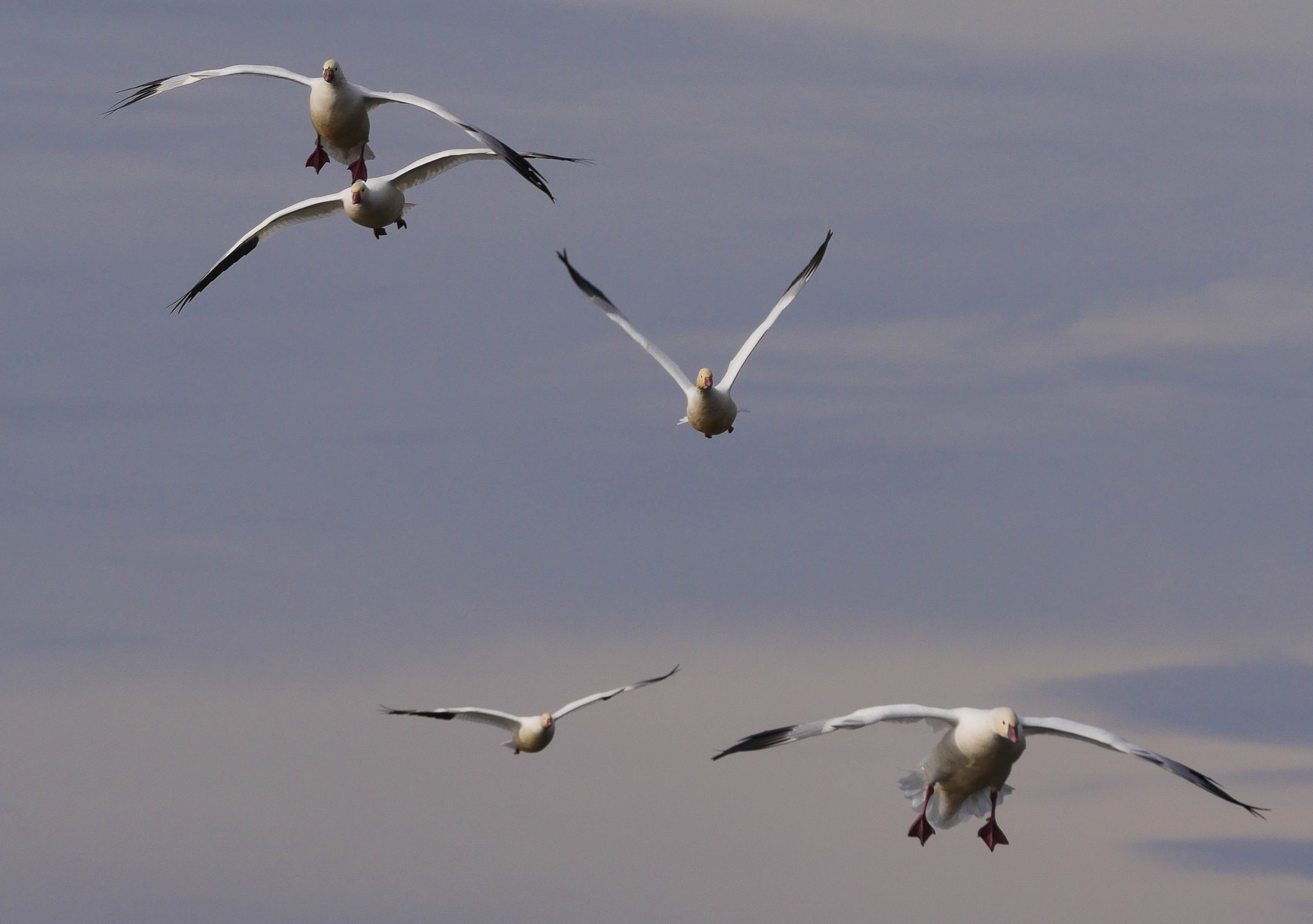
[164,148,598,314]
[555,227,835,439]
[379,665,681,754]
[712,703,1271,855]
[99,60,558,206]
